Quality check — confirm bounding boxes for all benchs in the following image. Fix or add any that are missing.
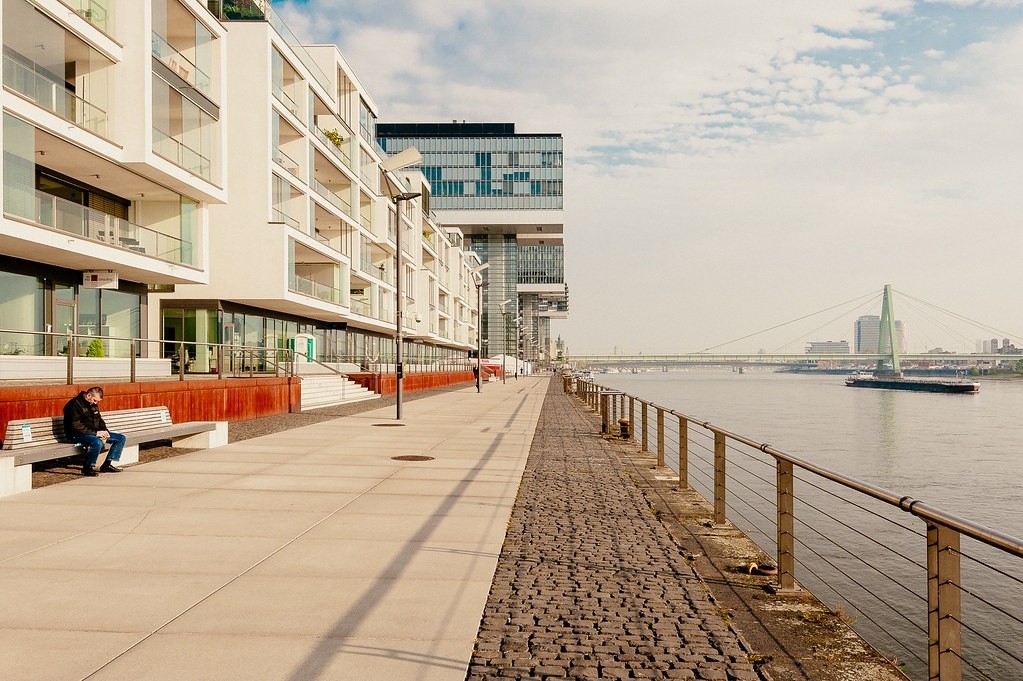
[0,404,228,505]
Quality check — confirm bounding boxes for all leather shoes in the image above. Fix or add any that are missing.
[100,464,123,472]
[81,466,98,476]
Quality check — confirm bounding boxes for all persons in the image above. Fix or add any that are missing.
[62,386,126,477]
[473,363,478,385]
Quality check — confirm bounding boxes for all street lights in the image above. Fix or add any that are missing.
[470,262,491,393]
[499,299,512,383]
[378,145,426,419]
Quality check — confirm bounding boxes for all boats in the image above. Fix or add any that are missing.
[843,376,980,393]
